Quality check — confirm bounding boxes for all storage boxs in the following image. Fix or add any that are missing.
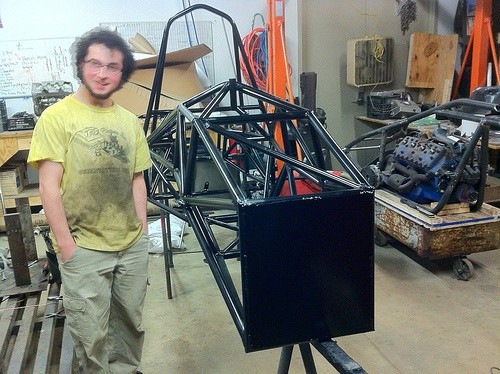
[107,61,225,149]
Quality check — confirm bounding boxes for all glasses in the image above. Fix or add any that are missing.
[85,58,124,74]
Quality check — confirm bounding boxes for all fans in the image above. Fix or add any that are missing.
[346,36,394,88]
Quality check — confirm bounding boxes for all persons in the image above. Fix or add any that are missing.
[25,23,155,374]
